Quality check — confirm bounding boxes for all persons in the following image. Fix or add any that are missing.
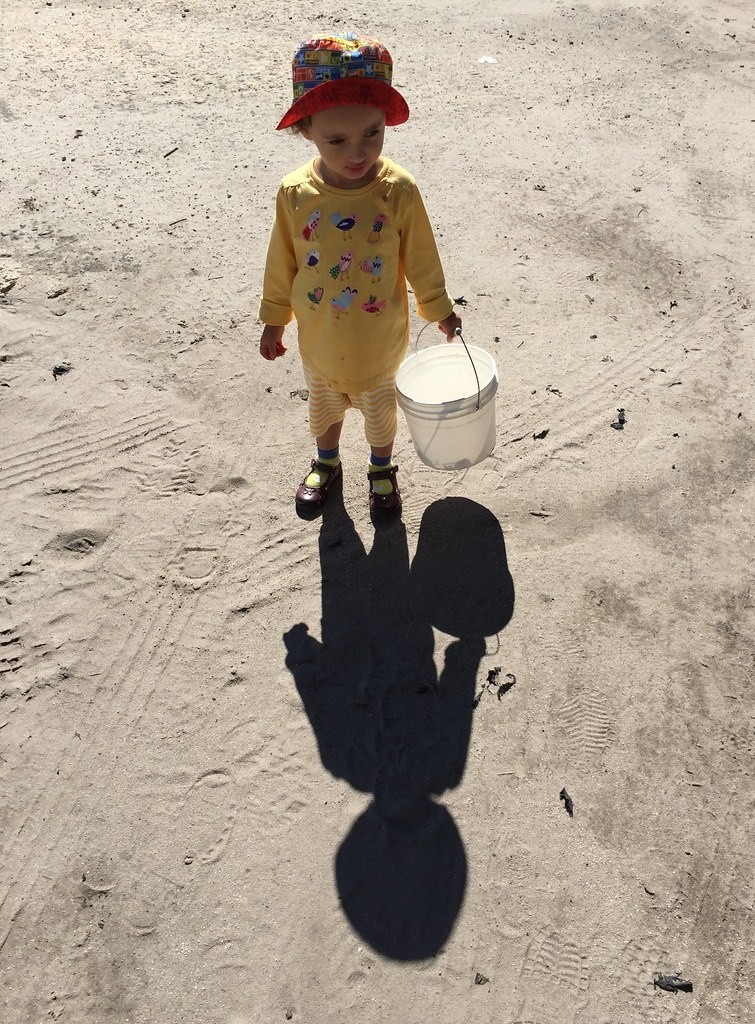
[259,33,462,517]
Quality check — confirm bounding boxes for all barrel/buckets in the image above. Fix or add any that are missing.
[395,322,499,471]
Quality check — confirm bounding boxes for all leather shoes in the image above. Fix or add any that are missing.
[295,459,342,508]
[367,465,403,515]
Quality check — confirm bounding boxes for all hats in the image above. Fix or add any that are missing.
[275,33,409,130]
